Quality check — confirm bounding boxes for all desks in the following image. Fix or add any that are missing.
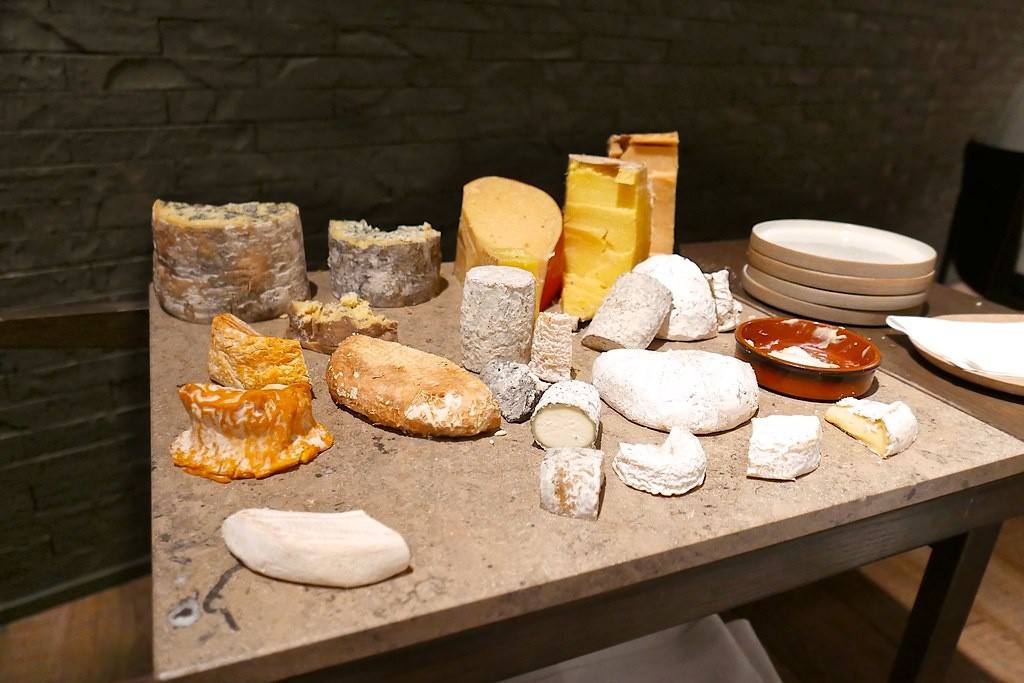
[149,237,1024,682]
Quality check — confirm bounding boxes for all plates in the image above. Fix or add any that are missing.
[743,219,938,325]
[885,314,1024,398]
[734,317,882,402]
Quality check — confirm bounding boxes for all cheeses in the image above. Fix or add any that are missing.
[151,133,921,588]
